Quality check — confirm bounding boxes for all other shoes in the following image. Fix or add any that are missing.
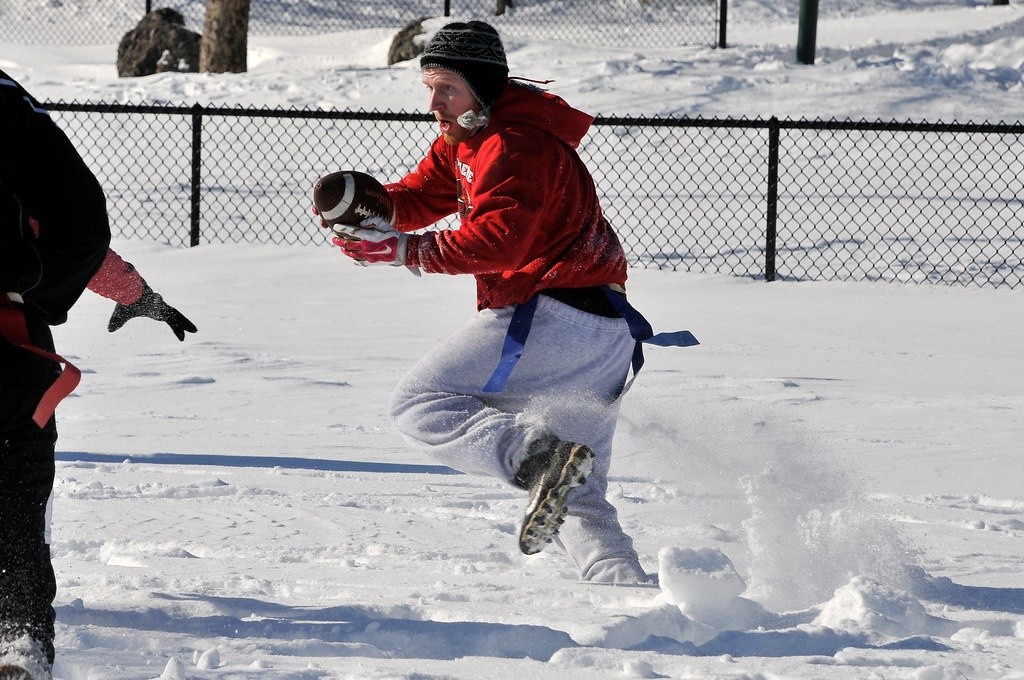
[0,636,55,680]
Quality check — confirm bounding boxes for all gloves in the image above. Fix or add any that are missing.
[312,205,329,229]
[331,216,407,268]
[108,285,198,341]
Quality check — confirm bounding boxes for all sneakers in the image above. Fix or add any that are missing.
[513,436,596,555]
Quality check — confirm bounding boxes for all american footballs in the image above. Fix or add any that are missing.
[314,170,396,235]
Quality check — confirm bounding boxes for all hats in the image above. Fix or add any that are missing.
[420,21,509,118]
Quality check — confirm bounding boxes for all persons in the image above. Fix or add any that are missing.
[313,20,700,582]
[0,70,198,679]
